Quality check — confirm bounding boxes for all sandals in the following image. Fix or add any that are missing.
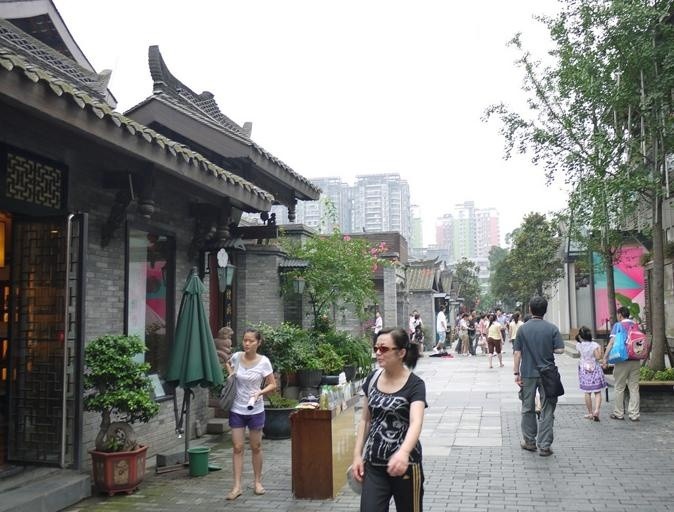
[226,486,242,500]
[584,413,600,422]
[254,483,265,495]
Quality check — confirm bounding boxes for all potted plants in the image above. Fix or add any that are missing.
[259,395,302,442]
[269,320,375,392]
[82,332,162,497]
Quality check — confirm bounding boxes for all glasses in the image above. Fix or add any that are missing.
[373,345,398,353]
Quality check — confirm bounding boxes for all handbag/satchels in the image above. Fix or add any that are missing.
[218,374,238,412]
[540,366,564,397]
[477,336,488,349]
[344,463,363,495]
[608,331,629,364]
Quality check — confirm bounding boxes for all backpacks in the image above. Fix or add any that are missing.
[626,327,648,361]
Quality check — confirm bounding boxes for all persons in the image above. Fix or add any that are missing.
[575,326,608,421]
[371,311,383,339]
[224,328,277,501]
[353,326,428,512]
[602,306,642,422]
[513,295,565,458]
[408,305,531,369]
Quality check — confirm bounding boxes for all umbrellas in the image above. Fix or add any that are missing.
[161,264,224,463]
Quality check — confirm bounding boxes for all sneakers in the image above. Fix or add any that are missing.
[538,448,552,456]
[520,440,537,451]
[610,413,625,420]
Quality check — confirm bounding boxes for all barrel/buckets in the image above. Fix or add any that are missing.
[188,446,211,477]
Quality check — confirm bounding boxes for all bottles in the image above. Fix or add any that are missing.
[320,372,350,410]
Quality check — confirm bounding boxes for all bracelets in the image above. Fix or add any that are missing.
[514,372,519,376]
[532,316,542,319]
